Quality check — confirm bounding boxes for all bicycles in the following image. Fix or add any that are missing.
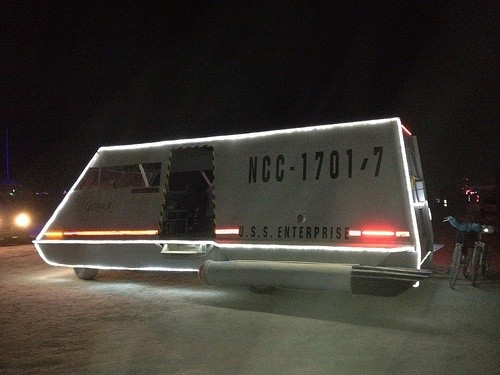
[462,223,498,287]
[442,216,482,289]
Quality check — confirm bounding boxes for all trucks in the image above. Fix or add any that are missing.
[30,117,452,297]
